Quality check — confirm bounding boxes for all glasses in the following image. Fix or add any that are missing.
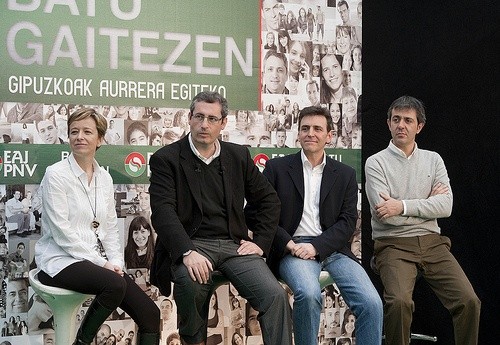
[191,114,223,123]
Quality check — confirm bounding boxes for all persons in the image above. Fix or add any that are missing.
[148,90,294,345]
[365,96,481,345]
[0,101,271,148]
[244,106,384,345]
[33,107,160,345]
[0,184,180,345]
[261,0,362,149]
[351,231,362,264]
[207,282,356,345]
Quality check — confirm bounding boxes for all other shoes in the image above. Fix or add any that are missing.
[17,231,32,238]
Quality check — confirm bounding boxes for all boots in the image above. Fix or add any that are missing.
[138,333,160,345]
[72,297,114,345]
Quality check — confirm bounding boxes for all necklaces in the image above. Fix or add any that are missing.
[77,175,100,228]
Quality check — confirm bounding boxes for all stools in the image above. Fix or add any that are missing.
[28,268,96,345]
[278,271,335,296]
[370,256,437,343]
